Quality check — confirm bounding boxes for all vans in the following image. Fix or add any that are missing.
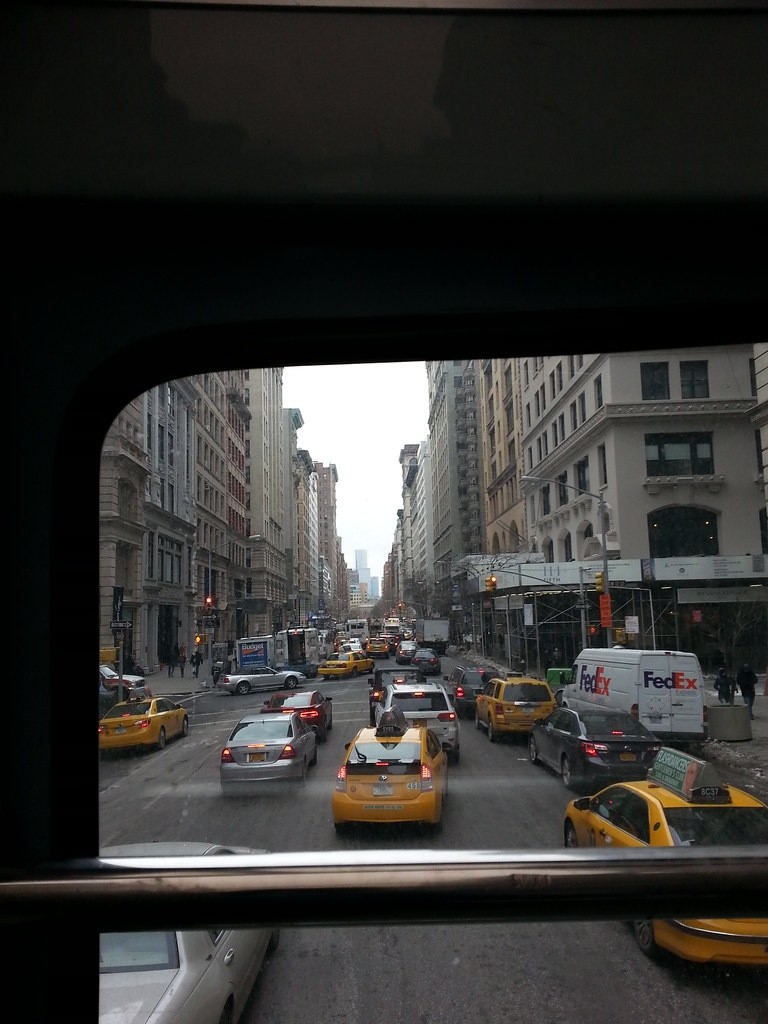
[560,648,709,750]
[474,677,557,741]
[443,664,501,720]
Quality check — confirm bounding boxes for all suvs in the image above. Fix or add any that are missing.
[369,681,461,763]
[369,667,428,728]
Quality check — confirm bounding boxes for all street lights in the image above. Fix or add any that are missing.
[201,534,261,688]
[520,475,613,648]
[436,560,485,657]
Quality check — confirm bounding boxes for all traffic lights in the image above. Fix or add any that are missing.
[485,577,492,593]
[491,576,497,593]
[594,571,605,594]
[589,627,600,634]
[205,597,212,603]
[195,636,201,643]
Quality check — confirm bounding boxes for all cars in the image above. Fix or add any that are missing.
[99,840,282,1023]
[527,706,665,796]
[562,746,768,970]
[98,686,188,754]
[331,705,454,836]
[260,689,333,743]
[220,712,318,790]
[99,664,146,702]
[216,616,449,695]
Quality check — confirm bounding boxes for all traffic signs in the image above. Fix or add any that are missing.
[110,620,133,629]
[574,603,592,611]
[202,614,218,620]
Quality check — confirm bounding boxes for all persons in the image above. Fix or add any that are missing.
[124,651,135,675]
[498,634,504,648]
[714,668,739,703]
[543,649,553,677]
[737,664,758,720]
[362,642,367,649]
[166,642,203,678]
[567,656,574,668]
[553,648,561,666]
